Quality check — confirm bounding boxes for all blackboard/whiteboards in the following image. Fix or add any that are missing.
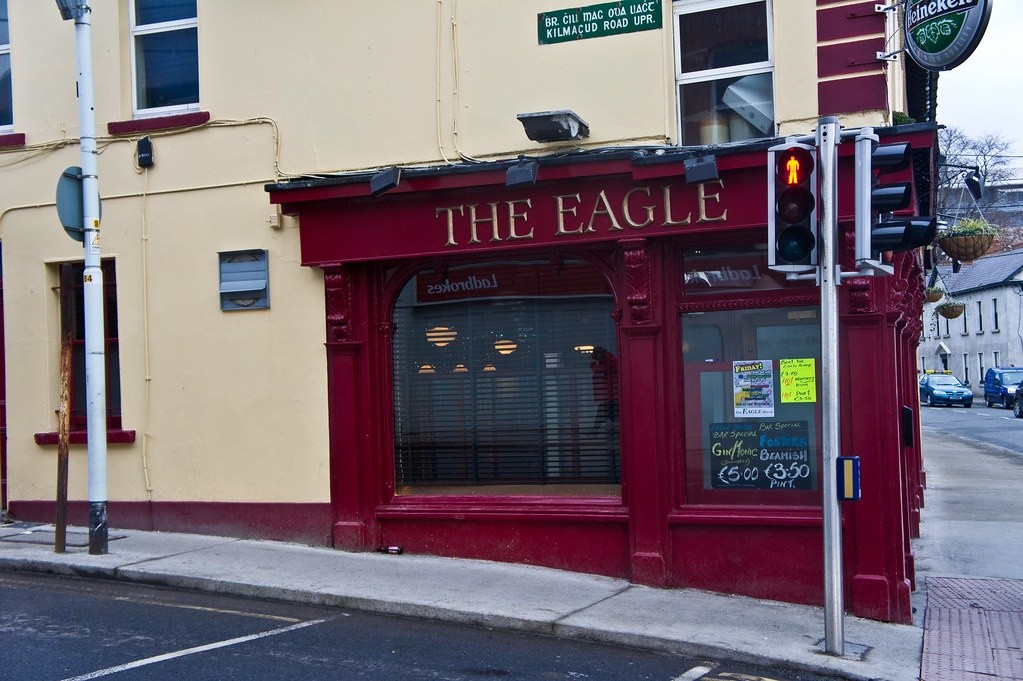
[710,421,812,488]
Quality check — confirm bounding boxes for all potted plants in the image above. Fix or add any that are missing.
[929,301,964,332]
[926,286,946,302]
[935,217,999,262]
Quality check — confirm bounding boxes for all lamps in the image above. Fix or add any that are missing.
[453,362,469,372]
[494,338,518,355]
[417,364,436,373]
[683,151,719,187]
[515,110,591,144]
[482,363,497,371]
[506,155,539,190]
[425,326,457,346]
[938,162,985,202]
[370,165,401,198]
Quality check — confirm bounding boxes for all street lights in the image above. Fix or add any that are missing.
[53,0,109,556]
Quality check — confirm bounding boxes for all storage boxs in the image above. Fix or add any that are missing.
[722,73,774,135]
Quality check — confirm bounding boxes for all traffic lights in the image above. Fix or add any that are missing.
[875,216,938,254]
[767,143,818,272]
[856,134,912,278]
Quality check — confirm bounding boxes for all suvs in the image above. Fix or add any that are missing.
[984,367,1023,409]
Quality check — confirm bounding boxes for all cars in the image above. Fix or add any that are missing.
[1013,380,1023,418]
[919,370,973,408]
